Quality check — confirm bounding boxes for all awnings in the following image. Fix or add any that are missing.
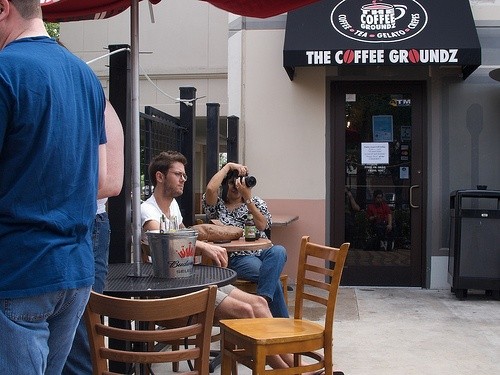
[282,0,481,82]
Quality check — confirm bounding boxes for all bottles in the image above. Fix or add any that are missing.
[245,211,256,241]
[160,213,178,232]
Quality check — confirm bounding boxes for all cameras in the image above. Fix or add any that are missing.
[232,170,257,189]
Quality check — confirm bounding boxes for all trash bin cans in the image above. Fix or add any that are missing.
[447,185,500,300]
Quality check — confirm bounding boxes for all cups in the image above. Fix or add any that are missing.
[256,233,260,239]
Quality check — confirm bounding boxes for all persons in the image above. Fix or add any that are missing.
[345,188,360,248]
[139,151,344,375]
[0,0,108,375]
[62,100,124,375]
[367,189,393,252]
[202,163,289,319]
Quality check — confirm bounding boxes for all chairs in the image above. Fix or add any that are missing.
[84,284,217,375]
[218,235,350,375]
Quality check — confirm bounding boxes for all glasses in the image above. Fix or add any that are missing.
[164,171,188,181]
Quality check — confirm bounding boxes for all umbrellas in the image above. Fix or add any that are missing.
[40,0,318,262]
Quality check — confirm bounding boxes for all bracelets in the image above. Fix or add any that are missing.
[244,198,253,204]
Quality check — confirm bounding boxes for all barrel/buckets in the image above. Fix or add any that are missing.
[145,229,198,279]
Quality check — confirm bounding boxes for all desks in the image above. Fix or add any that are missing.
[271,215,299,291]
[102,261,237,375]
[214,237,272,251]
